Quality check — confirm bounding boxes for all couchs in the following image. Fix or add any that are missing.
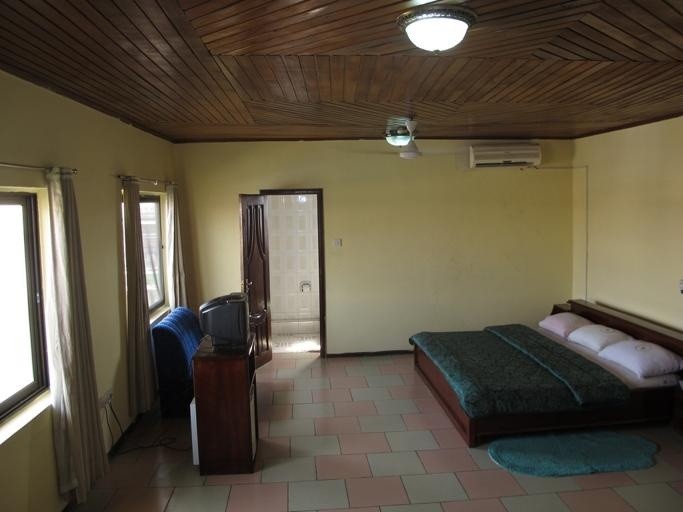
[152,307,205,420]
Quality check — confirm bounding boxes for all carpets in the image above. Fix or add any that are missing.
[488,430,658,477]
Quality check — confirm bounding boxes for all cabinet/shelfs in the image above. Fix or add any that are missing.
[191,333,260,476]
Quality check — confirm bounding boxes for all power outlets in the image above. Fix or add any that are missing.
[99,390,115,409]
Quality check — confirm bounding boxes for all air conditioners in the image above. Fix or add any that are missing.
[469,143,542,168]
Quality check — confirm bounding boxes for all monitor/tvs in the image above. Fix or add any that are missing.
[198,292,253,347]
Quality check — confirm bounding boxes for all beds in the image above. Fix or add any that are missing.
[409,299,683,448]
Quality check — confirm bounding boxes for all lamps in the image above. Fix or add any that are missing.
[383,129,417,147]
[396,4,478,53]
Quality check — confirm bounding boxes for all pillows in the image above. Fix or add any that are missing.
[567,323,635,351]
[538,312,595,337]
[598,339,683,379]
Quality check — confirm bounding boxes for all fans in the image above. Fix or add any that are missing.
[346,114,470,161]
